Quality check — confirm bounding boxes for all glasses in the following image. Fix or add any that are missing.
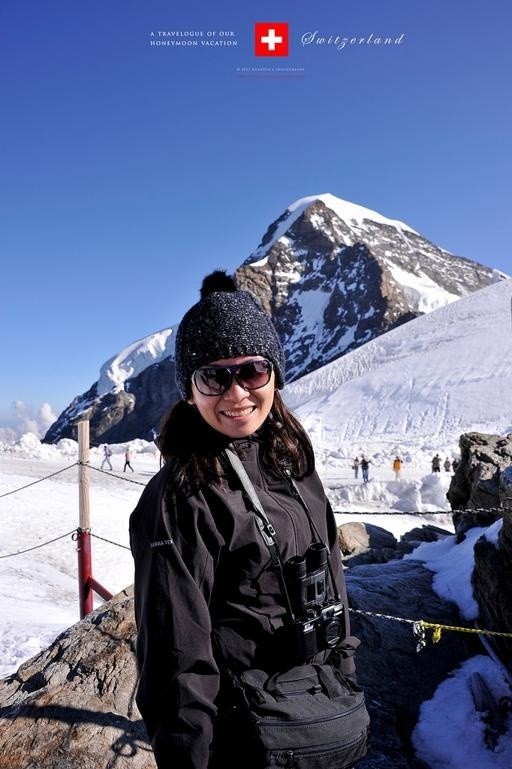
[185,357,275,399]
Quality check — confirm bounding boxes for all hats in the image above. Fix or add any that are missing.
[173,287,290,402]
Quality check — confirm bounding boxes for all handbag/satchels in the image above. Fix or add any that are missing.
[228,651,370,769]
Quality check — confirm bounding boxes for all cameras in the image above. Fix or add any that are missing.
[293,598,346,661]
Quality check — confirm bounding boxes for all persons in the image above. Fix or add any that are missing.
[392,455,403,481]
[431,454,442,472]
[352,456,361,478]
[128,268,369,769]
[122,447,135,473]
[443,456,451,472]
[99,444,114,470]
[451,458,459,472]
[359,454,371,483]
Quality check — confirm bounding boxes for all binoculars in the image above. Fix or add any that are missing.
[283,543,346,661]
